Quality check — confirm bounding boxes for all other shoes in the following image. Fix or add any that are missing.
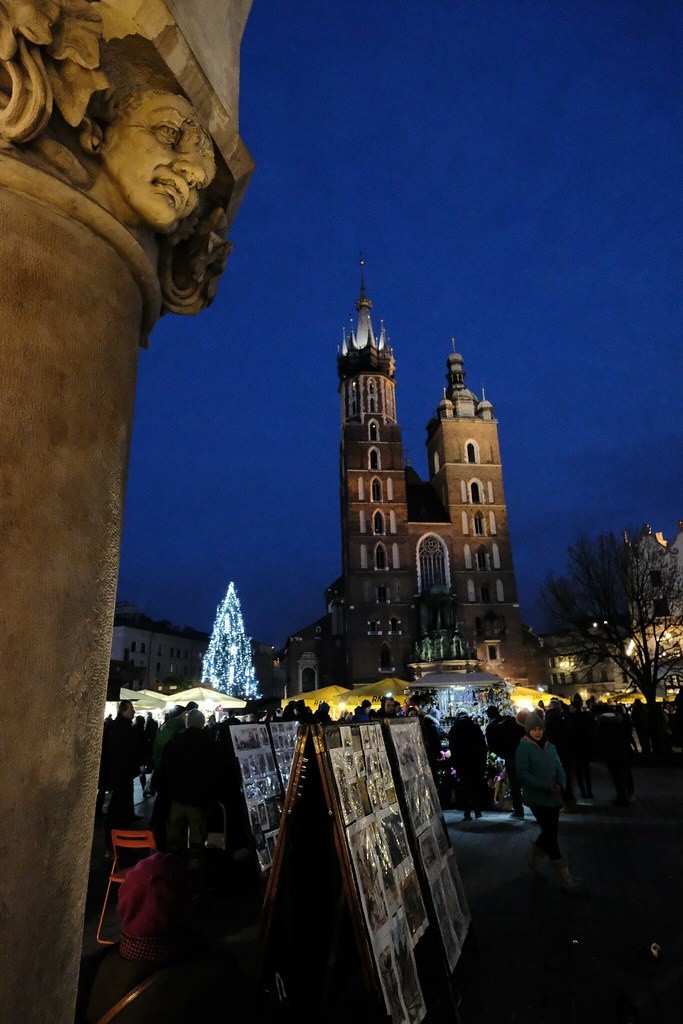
[512,812,524,819]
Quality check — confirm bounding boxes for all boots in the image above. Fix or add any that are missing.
[527,845,553,879]
[551,859,577,894]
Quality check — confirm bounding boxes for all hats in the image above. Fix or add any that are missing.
[524,715,544,733]
[115,852,188,935]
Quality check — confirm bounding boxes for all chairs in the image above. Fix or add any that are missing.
[95,829,159,945]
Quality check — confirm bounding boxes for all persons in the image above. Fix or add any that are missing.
[53,33,234,314]
[86,688,683,1024]
